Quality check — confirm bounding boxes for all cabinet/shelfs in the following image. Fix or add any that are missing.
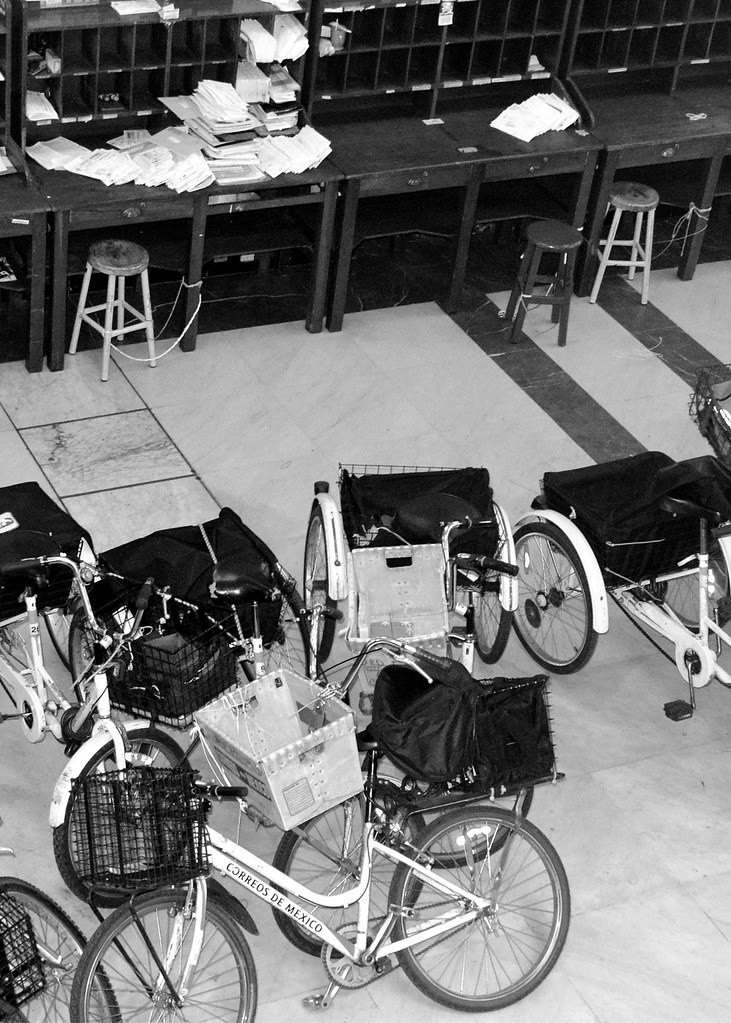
[0,0,731,147]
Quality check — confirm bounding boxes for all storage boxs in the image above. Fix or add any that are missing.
[192,668,364,831]
[346,543,452,690]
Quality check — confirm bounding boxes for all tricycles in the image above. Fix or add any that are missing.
[302,463,536,870]
[509,363,731,723]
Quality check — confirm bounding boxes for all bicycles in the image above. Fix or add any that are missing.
[1,520,573,1023]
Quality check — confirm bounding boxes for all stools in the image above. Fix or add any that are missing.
[66,238,160,382]
[588,181,662,306]
[504,218,584,347]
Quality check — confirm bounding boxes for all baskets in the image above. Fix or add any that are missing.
[687,360,731,468]
[428,675,558,794]
[69,765,212,893]
[0,890,46,1022]
[75,587,242,729]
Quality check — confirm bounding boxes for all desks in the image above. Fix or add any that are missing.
[0,154,50,376]
[44,125,345,372]
[321,102,603,335]
[574,85,731,299]
